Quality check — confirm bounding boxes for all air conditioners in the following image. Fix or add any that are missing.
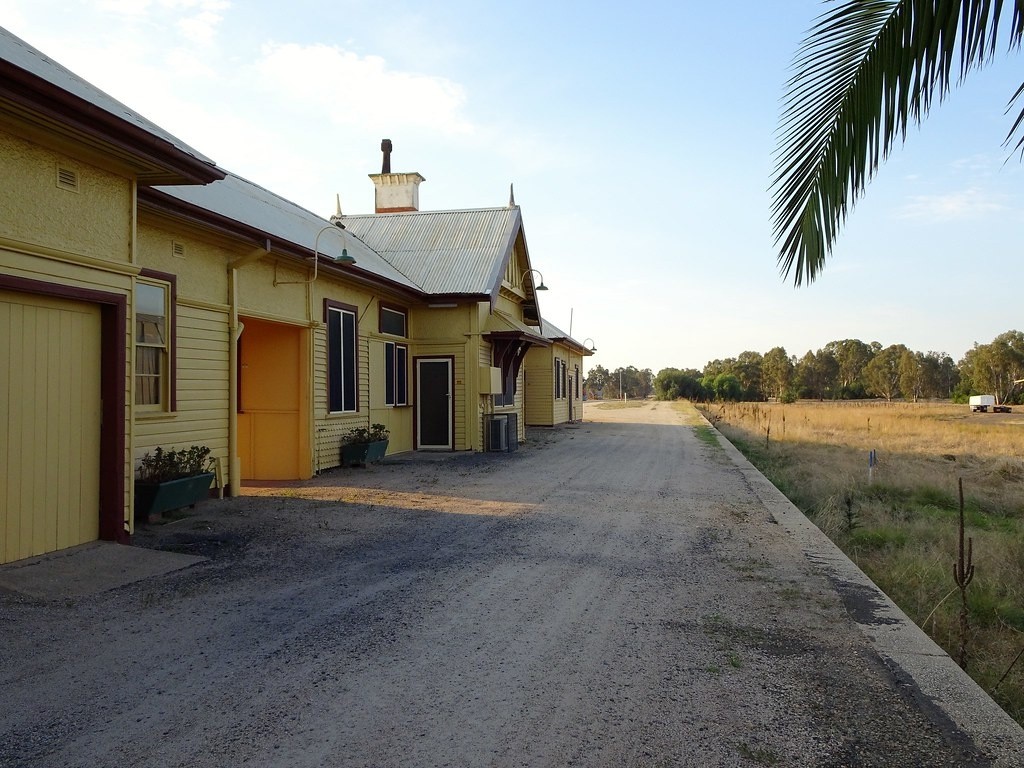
[487,418,508,451]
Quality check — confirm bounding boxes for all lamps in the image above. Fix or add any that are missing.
[274,225,356,286]
[581,338,597,355]
[511,269,548,290]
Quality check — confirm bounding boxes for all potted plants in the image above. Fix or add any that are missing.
[134,445,215,514]
[338,423,390,465]
